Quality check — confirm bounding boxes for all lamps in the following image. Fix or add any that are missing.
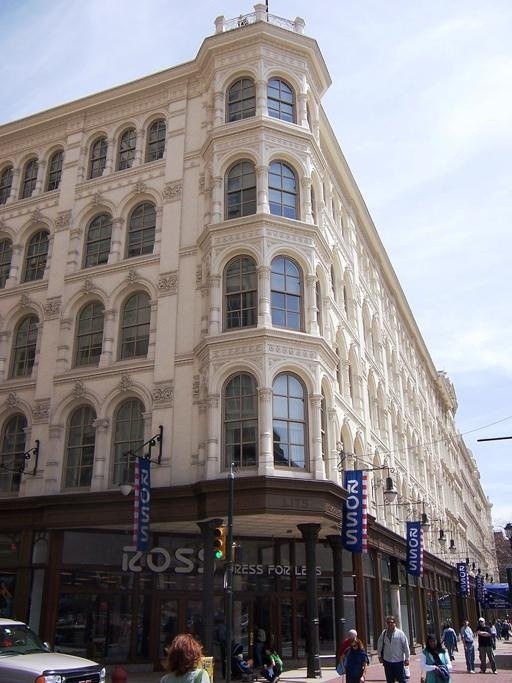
[119,424,164,497]
[363,465,494,584]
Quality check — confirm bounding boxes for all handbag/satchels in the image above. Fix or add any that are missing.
[336,656,348,675]
[402,665,411,681]
[381,646,384,661]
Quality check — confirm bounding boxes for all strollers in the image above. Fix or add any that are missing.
[231,642,257,680]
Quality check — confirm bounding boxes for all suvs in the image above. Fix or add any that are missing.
[0,619,106,682]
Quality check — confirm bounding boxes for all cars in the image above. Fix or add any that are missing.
[49,624,89,658]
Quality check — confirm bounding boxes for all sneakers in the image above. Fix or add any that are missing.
[468,670,477,674]
[493,670,498,674]
[274,677,280,683]
[480,671,487,673]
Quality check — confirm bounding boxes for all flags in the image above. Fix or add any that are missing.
[456,562,471,599]
[339,469,369,554]
[406,521,423,578]
[474,574,485,603]
[131,457,152,550]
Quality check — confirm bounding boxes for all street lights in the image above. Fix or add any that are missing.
[503,523,512,547]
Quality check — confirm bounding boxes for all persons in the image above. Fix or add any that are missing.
[420,632,453,682]
[476,616,498,674]
[261,646,284,683]
[337,628,365,682]
[440,622,458,661]
[344,638,369,682]
[377,614,411,683]
[158,631,211,682]
[460,619,477,674]
[488,617,512,643]
[235,653,254,674]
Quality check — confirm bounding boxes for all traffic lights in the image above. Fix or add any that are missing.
[214,527,227,560]
